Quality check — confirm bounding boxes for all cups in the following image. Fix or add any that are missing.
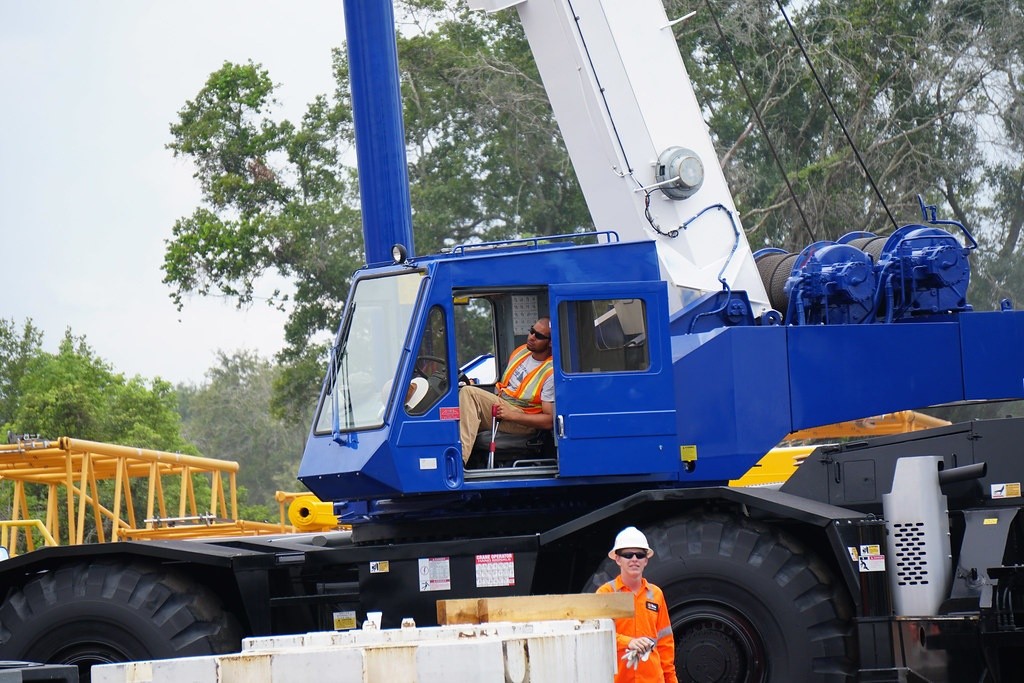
[367,612,382,630]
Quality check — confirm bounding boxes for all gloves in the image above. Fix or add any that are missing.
[620,637,655,671]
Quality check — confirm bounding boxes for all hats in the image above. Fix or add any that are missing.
[608,527,654,560]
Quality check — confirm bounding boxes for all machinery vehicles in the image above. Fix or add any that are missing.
[0,2,1024,683]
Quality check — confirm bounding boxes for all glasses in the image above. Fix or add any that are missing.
[617,552,649,559]
[529,325,550,341]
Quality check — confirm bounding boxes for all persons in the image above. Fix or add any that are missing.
[595,524,678,683]
[458,316,555,465]
